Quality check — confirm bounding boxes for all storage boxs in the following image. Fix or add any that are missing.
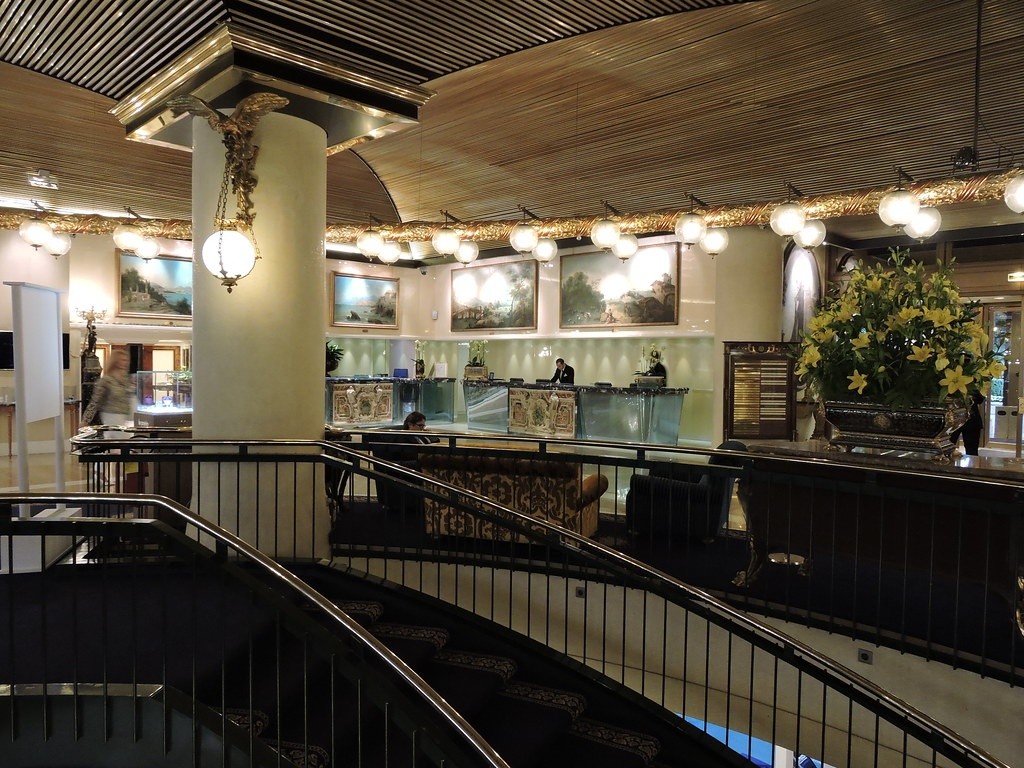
[136,371,193,414]
[636,375,664,388]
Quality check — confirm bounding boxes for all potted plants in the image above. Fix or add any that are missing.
[326,336,345,377]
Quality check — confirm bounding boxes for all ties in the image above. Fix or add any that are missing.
[419,436,429,444]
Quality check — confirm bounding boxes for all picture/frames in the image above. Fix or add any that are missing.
[559,240,680,329]
[114,246,193,321]
[329,269,400,331]
[449,261,539,333]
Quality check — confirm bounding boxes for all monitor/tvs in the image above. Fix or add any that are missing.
[536,379,552,383]
[594,382,612,387]
[510,378,525,382]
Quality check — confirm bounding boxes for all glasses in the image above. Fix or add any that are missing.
[413,422,426,429]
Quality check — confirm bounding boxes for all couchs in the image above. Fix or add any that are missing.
[625,439,747,546]
[374,422,423,510]
[415,450,610,548]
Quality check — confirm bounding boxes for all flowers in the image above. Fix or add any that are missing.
[466,338,488,367]
[413,339,427,361]
[788,245,1008,404]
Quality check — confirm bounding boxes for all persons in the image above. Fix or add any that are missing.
[950,388,988,456]
[550,359,574,384]
[376,412,440,546]
[79,350,134,488]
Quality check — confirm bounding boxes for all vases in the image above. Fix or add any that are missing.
[826,391,977,459]
[416,360,425,377]
[463,364,490,380]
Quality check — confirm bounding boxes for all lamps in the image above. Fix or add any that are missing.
[17,199,52,251]
[46,232,74,262]
[590,198,621,252]
[511,207,540,255]
[457,240,479,268]
[876,168,921,233]
[796,219,827,251]
[532,235,559,265]
[200,133,263,293]
[770,183,805,243]
[357,212,384,263]
[431,208,461,257]
[905,202,943,243]
[380,239,402,268]
[699,227,730,260]
[112,205,145,252]
[1003,172,1024,214]
[613,234,639,263]
[135,234,163,263]
[674,194,708,250]
